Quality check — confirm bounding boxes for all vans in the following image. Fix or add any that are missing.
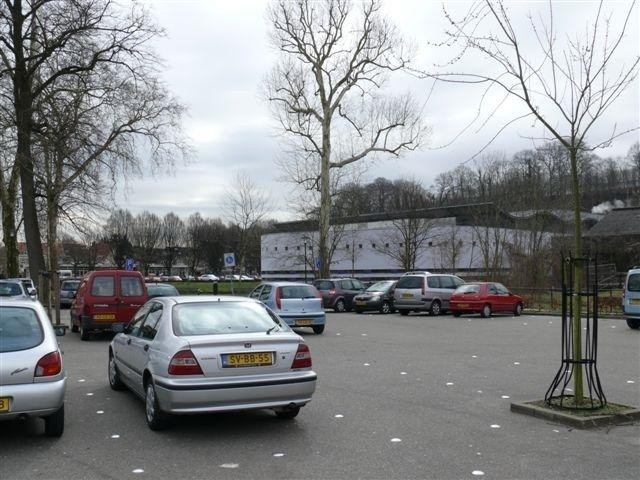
[67,269,148,340]
[618,267,640,329]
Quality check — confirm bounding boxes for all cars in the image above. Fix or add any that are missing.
[145,284,182,300]
[0,266,83,310]
[145,272,260,282]
[244,280,329,338]
[103,295,315,432]
[0,295,70,439]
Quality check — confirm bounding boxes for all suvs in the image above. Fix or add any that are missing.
[309,269,526,320]
